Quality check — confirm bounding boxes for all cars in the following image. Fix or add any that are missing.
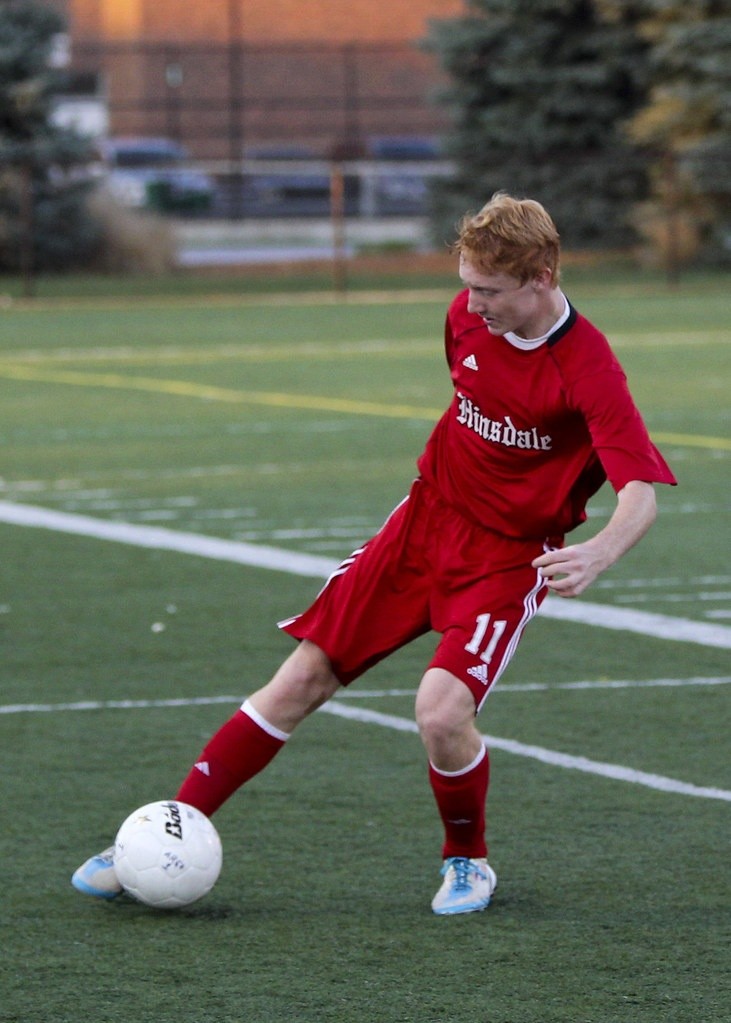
[96,133,217,210]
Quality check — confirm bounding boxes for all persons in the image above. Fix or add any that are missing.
[72,191,676,918]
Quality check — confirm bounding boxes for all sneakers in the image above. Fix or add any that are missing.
[71,847,122,896]
[430,857,498,915]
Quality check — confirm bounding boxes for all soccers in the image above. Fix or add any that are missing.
[113,800,225,910]
